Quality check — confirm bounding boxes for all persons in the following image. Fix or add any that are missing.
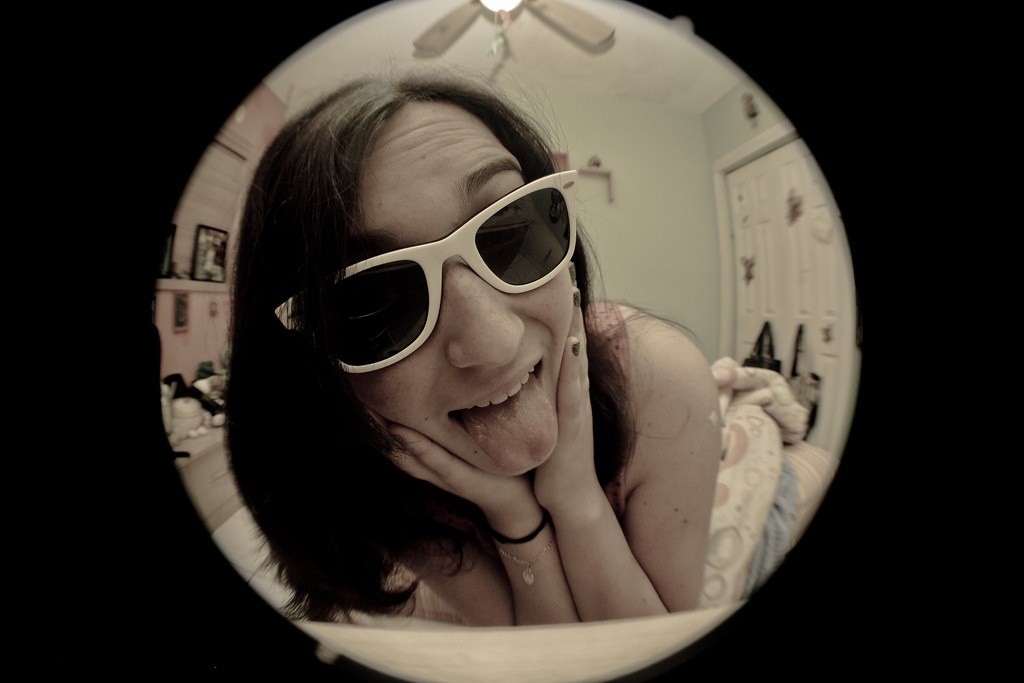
[205,241,226,280]
[223,76,723,620]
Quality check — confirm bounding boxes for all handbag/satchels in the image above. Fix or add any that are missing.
[740,320,781,374]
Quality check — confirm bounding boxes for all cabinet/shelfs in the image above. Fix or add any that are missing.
[726,137,862,459]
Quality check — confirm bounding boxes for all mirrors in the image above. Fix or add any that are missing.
[148,0,859,683]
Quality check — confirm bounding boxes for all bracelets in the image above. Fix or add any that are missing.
[492,517,548,545]
[499,536,554,585]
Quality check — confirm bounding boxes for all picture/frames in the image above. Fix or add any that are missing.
[189,223,228,283]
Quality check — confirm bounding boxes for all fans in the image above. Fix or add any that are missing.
[413,0,615,52]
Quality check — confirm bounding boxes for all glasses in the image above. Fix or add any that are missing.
[274,169,579,373]
